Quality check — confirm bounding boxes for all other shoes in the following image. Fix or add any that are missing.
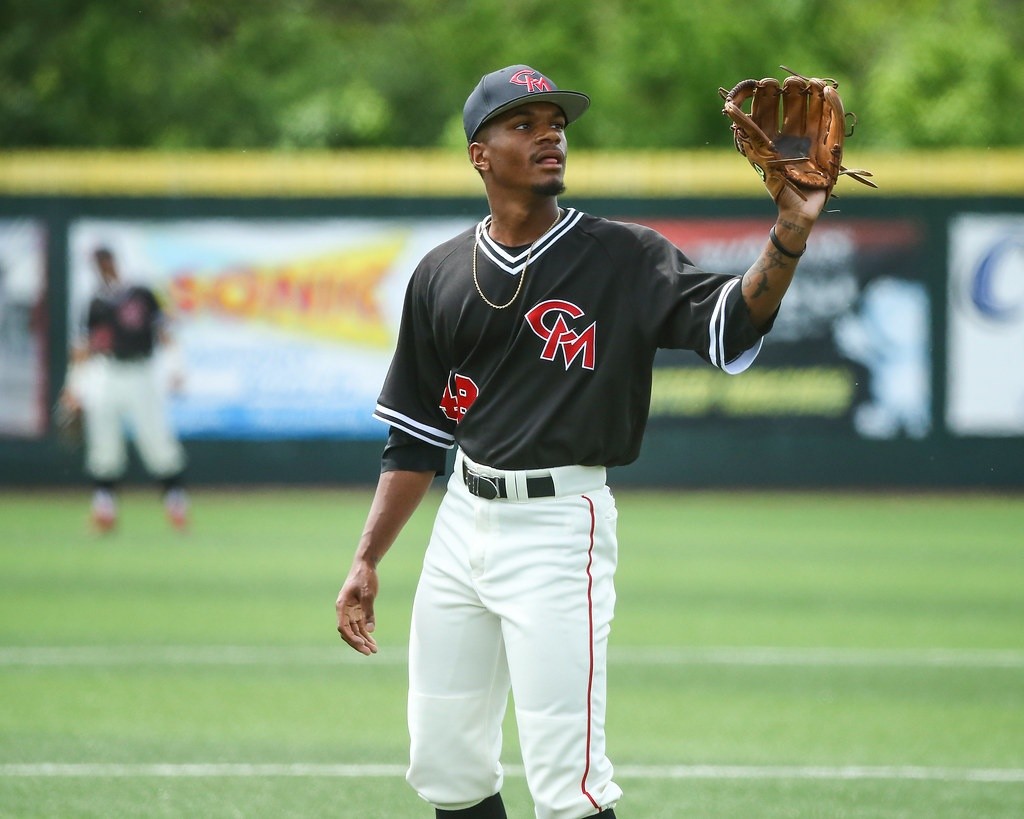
[166,486,188,527]
[89,493,114,522]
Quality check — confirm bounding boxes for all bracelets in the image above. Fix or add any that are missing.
[769,223,807,258]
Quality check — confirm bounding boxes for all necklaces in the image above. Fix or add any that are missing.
[471,210,561,311]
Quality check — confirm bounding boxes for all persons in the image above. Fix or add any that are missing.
[334,63,846,818]
[59,246,190,531]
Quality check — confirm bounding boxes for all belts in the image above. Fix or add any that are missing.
[460,462,554,500]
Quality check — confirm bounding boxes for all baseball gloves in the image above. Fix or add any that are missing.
[722,78,846,203]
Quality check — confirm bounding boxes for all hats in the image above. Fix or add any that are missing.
[462,65,591,145]
